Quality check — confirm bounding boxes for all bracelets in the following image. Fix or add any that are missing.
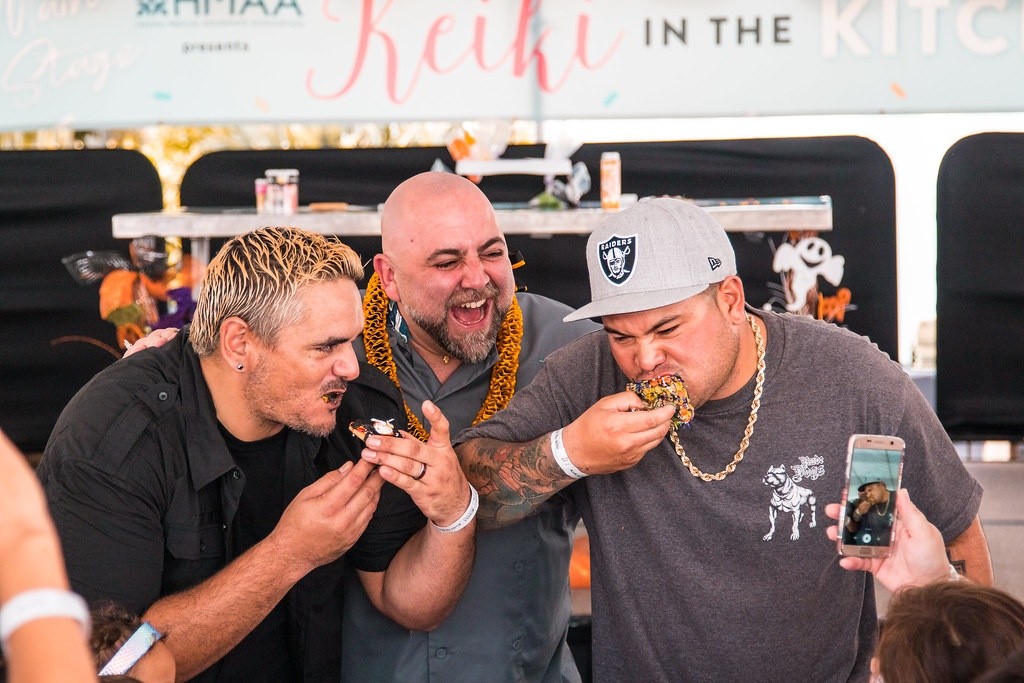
[0,585,91,642]
[855,509,862,516]
[845,517,850,525]
[429,482,479,533]
[551,428,587,478]
[949,566,958,581]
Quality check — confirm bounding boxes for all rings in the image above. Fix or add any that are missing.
[414,463,426,480]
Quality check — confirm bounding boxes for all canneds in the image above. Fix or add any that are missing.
[264,168,299,216]
[600,151,622,209]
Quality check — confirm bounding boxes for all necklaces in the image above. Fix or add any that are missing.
[669,312,766,482]
[414,340,450,364]
[876,491,890,516]
[364,271,523,444]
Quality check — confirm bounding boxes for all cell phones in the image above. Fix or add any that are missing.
[837,433,907,559]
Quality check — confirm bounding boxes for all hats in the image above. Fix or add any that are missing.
[857,477,886,491]
[562,196,737,323]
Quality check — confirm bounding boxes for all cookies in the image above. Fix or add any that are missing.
[349,419,403,442]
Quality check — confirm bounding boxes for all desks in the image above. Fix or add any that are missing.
[113,194,833,335]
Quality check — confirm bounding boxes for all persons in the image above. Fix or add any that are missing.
[37,227,476,683]
[126,170,604,683]
[845,476,896,546]
[825,488,1024,683]
[0,428,175,683]
[454,197,994,683]
